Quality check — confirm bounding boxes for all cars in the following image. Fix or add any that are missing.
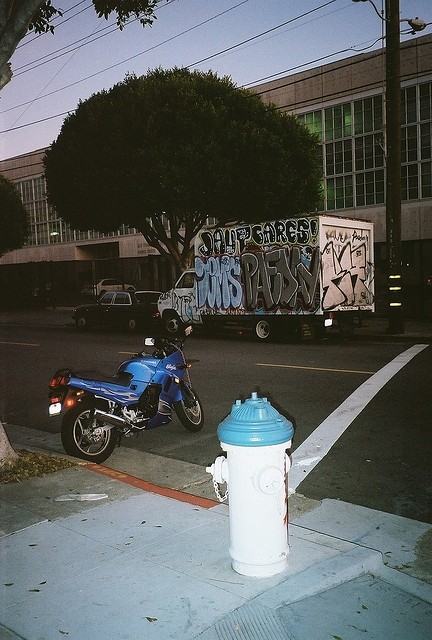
[92,278,136,297]
[72,291,163,333]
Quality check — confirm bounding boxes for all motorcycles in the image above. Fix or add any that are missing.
[48,324,204,464]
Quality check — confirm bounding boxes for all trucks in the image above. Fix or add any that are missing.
[157,215,376,343]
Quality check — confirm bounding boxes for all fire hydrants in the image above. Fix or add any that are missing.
[205,392,294,579]
